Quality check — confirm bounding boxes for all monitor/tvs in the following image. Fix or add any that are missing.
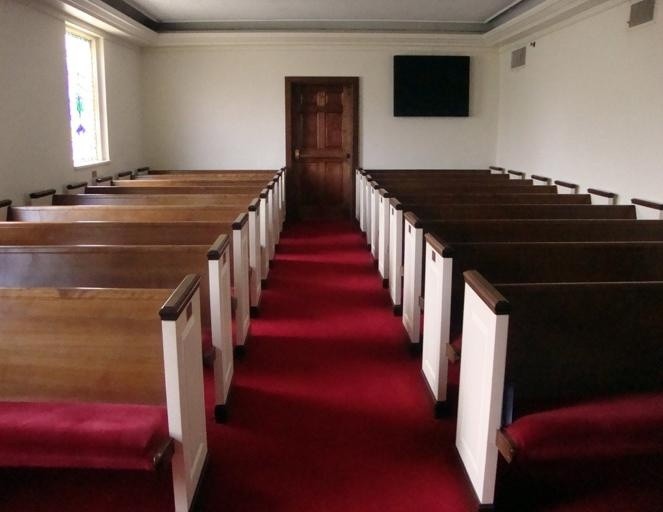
[392,54,472,119]
